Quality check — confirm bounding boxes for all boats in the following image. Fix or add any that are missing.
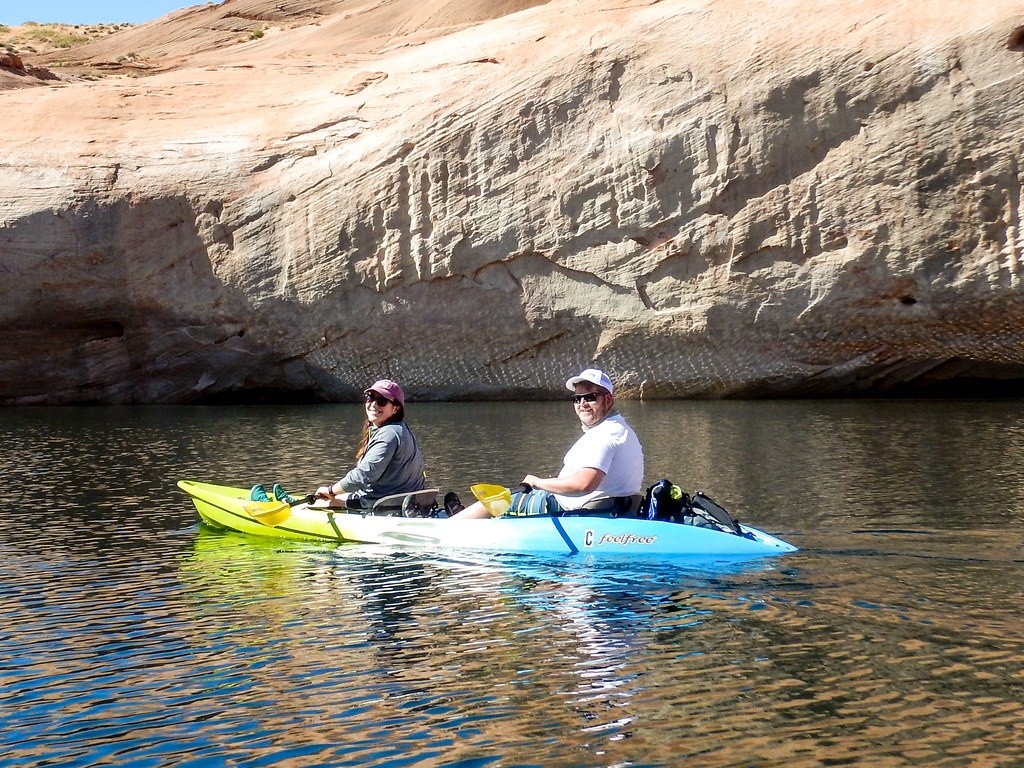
[175,478,802,568]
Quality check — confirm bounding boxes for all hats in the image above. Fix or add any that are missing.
[565,368,613,397]
[364,380,404,409]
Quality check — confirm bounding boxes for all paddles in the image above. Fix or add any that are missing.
[469,482,532,518]
[238,487,323,529]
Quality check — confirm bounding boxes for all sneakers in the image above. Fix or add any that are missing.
[273,484,296,503]
[250,484,270,502]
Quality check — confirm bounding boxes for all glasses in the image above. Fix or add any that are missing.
[365,393,395,407]
[570,392,607,403]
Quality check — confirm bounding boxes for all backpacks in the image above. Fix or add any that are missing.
[636,481,692,520]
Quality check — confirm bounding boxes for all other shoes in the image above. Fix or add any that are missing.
[402,495,426,518]
[444,492,465,517]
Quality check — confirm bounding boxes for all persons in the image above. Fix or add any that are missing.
[249,379,427,514]
[445,368,644,520]
